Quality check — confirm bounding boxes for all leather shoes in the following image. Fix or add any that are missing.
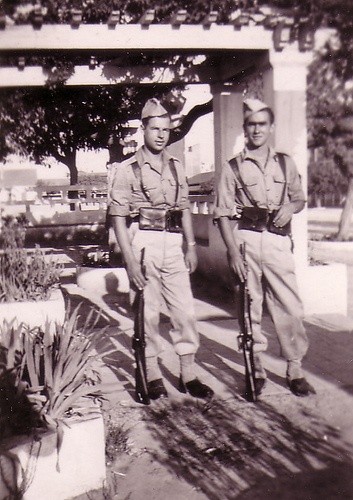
[178,375,216,401]
[286,375,318,398]
[146,379,169,400]
[248,378,267,396]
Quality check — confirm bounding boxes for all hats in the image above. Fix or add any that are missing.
[139,97,168,119]
[242,98,268,118]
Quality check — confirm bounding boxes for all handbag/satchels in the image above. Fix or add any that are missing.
[269,209,292,238]
[138,208,164,231]
[238,206,268,232]
[165,209,185,233]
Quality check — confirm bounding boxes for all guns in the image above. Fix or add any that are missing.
[237,241,257,401]
[131,247,150,405]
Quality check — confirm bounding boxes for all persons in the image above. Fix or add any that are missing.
[108,98,216,405]
[213,100,318,398]
